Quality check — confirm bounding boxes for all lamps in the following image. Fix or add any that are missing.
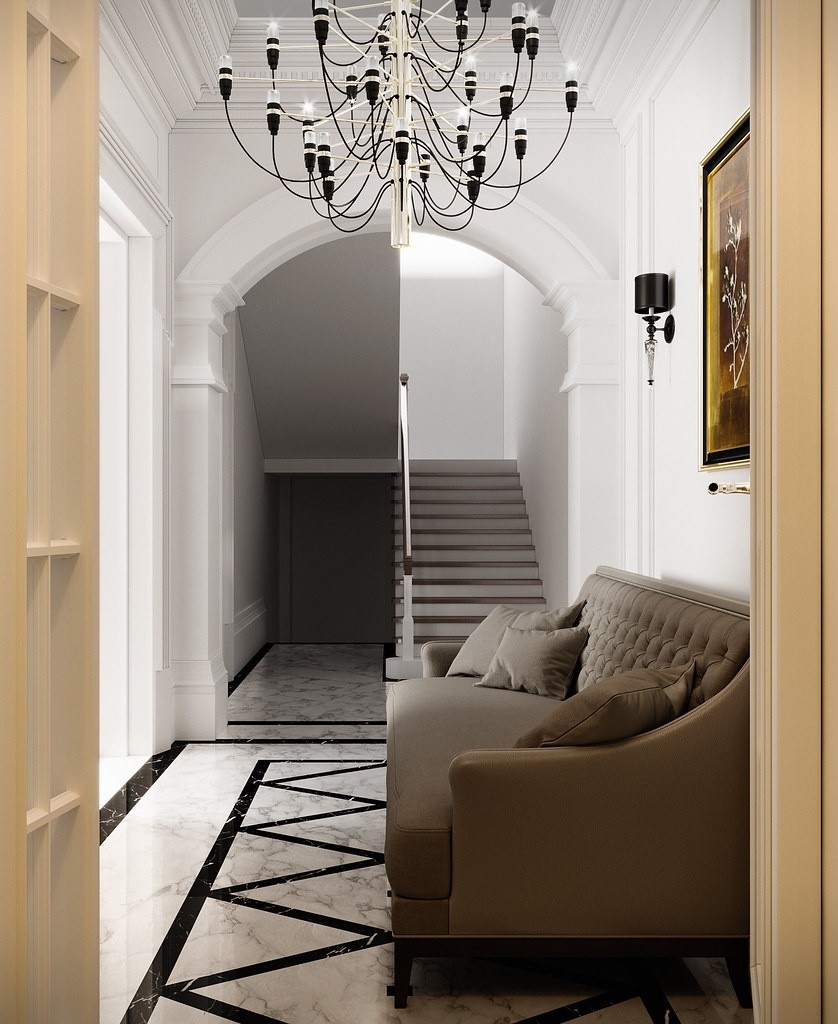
[219,0,578,248]
[635,273,675,385]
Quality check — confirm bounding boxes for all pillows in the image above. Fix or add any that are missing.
[512,657,695,748]
[474,626,590,701]
[445,600,587,678]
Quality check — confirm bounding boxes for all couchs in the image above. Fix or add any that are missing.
[386,566,750,1009]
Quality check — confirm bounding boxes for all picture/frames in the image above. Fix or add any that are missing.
[698,106,751,472]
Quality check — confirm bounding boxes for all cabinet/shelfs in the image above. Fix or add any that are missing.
[0,1,99,1024]
